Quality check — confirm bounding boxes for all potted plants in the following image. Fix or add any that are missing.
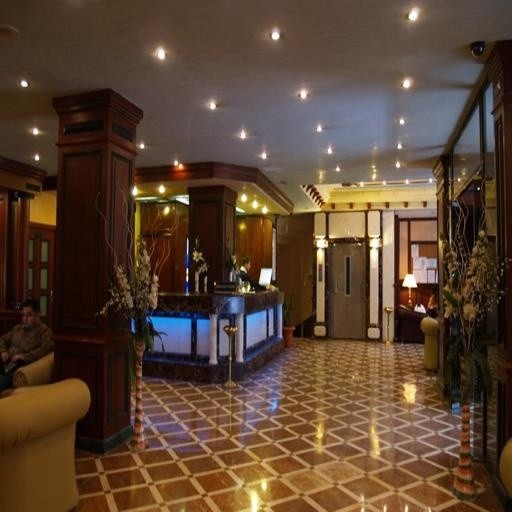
[282,293,296,348]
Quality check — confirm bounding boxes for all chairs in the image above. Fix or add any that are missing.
[420,316,439,369]
[397,307,429,343]
[0,378,91,512]
[11,352,55,388]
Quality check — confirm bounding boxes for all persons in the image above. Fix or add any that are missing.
[238,256,276,291]
[1,300,54,392]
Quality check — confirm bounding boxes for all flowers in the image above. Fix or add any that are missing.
[93,182,172,341]
[440,176,512,405]
[190,248,209,278]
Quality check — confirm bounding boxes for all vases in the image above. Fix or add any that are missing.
[194,271,199,294]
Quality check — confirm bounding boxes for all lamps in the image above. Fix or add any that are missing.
[401,274,418,309]
